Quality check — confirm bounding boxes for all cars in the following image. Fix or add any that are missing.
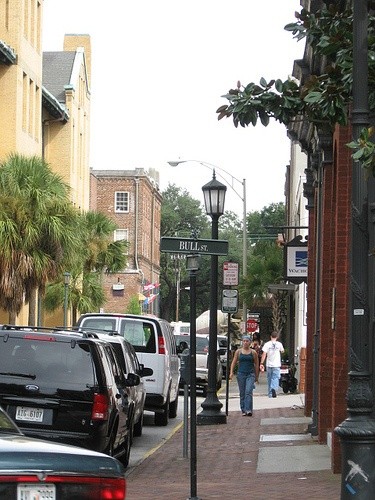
[216,336,234,379]
[0,407,126,500]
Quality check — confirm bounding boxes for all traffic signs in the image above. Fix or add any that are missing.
[160,237,229,256]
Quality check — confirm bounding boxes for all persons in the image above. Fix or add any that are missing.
[229,335,260,416]
[179,342,188,352]
[252,332,262,382]
[260,330,285,398]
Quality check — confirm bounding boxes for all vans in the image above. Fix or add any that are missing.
[73,311,181,425]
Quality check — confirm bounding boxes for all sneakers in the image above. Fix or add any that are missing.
[246,411,252,416]
[272,389,276,397]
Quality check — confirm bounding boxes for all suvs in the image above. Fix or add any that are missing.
[50,327,153,437]
[0,325,140,467]
[173,333,225,396]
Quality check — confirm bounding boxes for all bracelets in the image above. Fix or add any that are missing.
[259,363,265,365]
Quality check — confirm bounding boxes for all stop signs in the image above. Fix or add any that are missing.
[246,320,257,332]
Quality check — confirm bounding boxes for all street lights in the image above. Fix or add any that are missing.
[168,159,248,337]
[62,271,70,332]
[196,169,226,427]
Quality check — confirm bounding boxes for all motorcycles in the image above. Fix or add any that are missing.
[279,347,300,393]
